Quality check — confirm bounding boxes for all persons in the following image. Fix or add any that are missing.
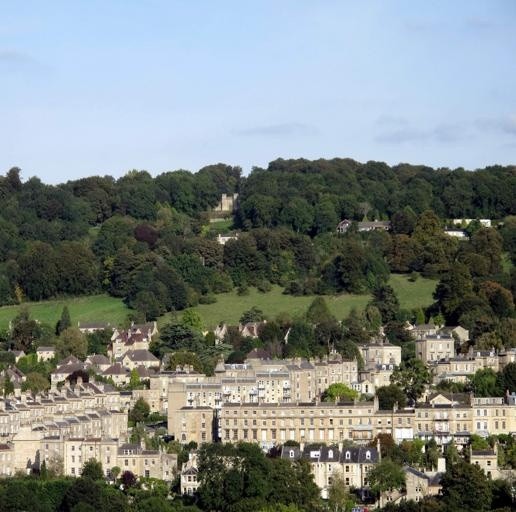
[351,505,369,512]
